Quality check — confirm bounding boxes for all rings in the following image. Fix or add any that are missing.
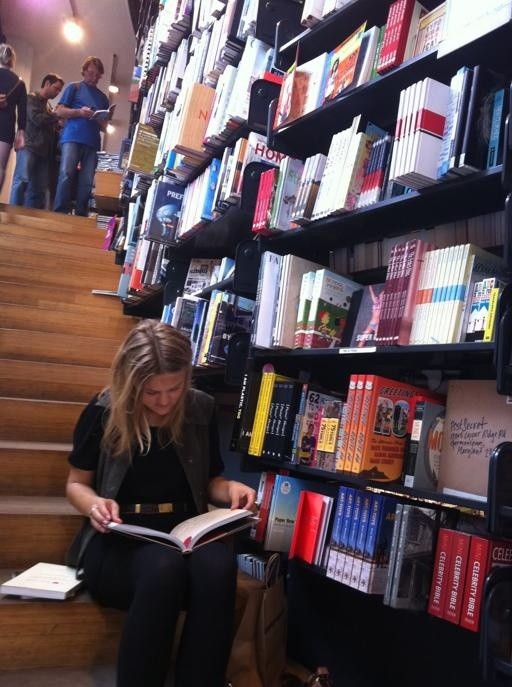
[89,503,99,514]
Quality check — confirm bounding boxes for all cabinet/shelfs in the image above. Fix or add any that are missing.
[108,0,512,687]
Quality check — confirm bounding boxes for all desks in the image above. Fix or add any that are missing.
[91,169,123,213]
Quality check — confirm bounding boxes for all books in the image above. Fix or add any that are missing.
[251,469,511,632]
[0,562,87,600]
[237,546,275,583]
[230,364,511,502]
[96,1,512,370]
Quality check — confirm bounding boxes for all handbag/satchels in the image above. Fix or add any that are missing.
[224,574,288,687]
[0,94,6,110]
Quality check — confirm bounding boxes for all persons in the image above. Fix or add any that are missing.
[9,74,65,209]
[65,318,257,687]
[56,56,109,217]
[0,43,28,197]
